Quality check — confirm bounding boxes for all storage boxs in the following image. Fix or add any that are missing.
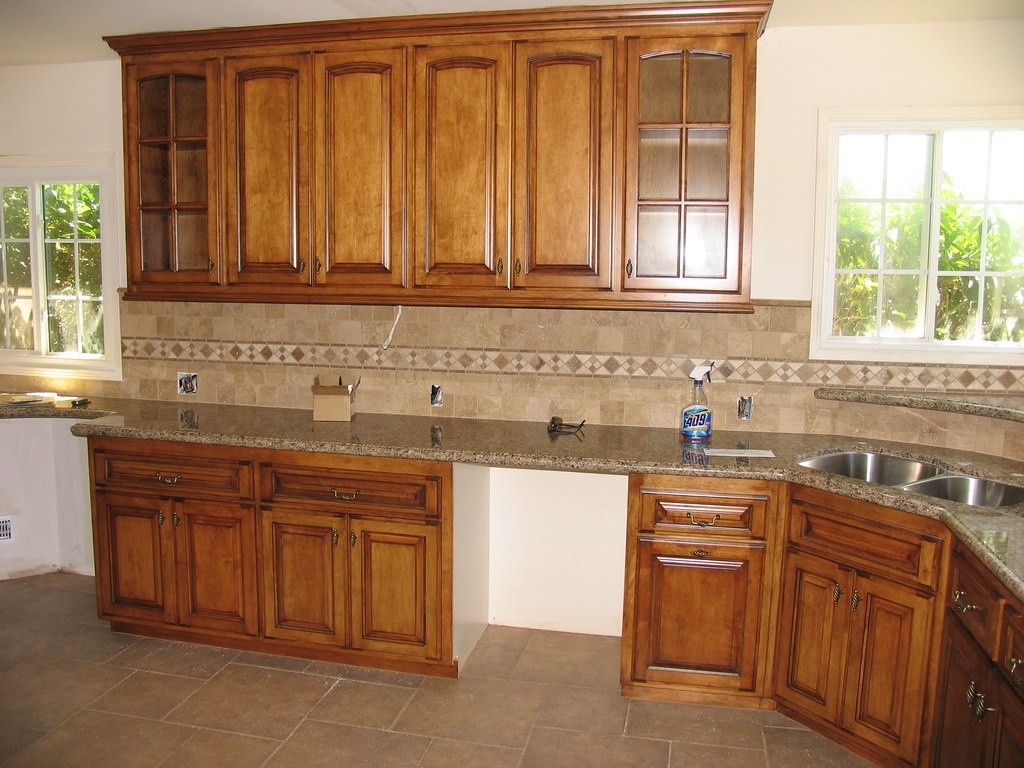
[310,374,363,422]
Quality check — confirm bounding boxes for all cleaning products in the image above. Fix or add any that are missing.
[680,364,714,436]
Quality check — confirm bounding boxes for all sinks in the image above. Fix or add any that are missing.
[795,444,945,487]
[901,472,1024,506]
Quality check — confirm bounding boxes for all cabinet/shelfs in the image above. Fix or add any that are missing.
[759,482,952,767]
[617,471,778,710]
[252,447,491,680]
[99,0,772,314]
[929,533,1024,768]
[86,436,261,651]
